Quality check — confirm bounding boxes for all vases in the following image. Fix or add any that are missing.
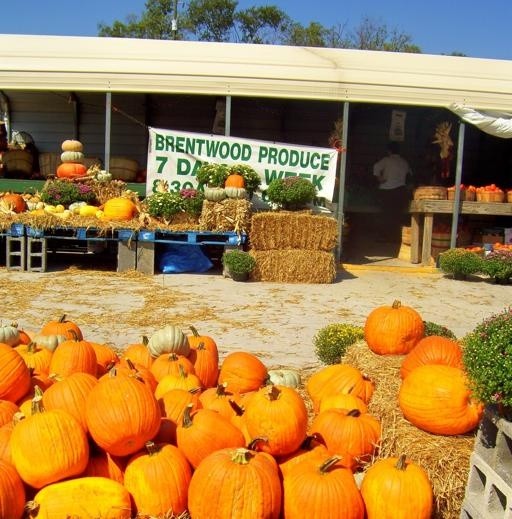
[283,202,306,211]
[498,402,512,416]
[495,276,509,284]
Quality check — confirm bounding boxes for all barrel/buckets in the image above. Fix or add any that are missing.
[2,150,140,181]
[400,184,512,248]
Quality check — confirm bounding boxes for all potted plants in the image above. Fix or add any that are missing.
[196,163,228,188]
[439,248,480,280]
[221,250,256,281]
[228,163,261,198]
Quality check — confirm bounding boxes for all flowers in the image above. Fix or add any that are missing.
[266,176,316,204]
[146,187,206,220]
[457,306,512,408]
[480,250,512,279]
[40,180,96,206]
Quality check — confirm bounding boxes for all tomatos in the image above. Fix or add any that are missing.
[447,183,512,194]
[463,247,482,251]
[496,242,512,250]
[432,223,451,233]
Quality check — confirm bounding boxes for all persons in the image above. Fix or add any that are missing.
[373,141,410,243]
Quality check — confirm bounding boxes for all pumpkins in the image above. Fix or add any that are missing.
[204,173,246,202]
[57,139,87,179]
[0,192,140,219]
[1,299,490,518]
[97,170,113,181]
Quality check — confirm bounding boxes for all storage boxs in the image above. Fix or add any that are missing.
[481,233,504,243]
[504,228,512,244]
